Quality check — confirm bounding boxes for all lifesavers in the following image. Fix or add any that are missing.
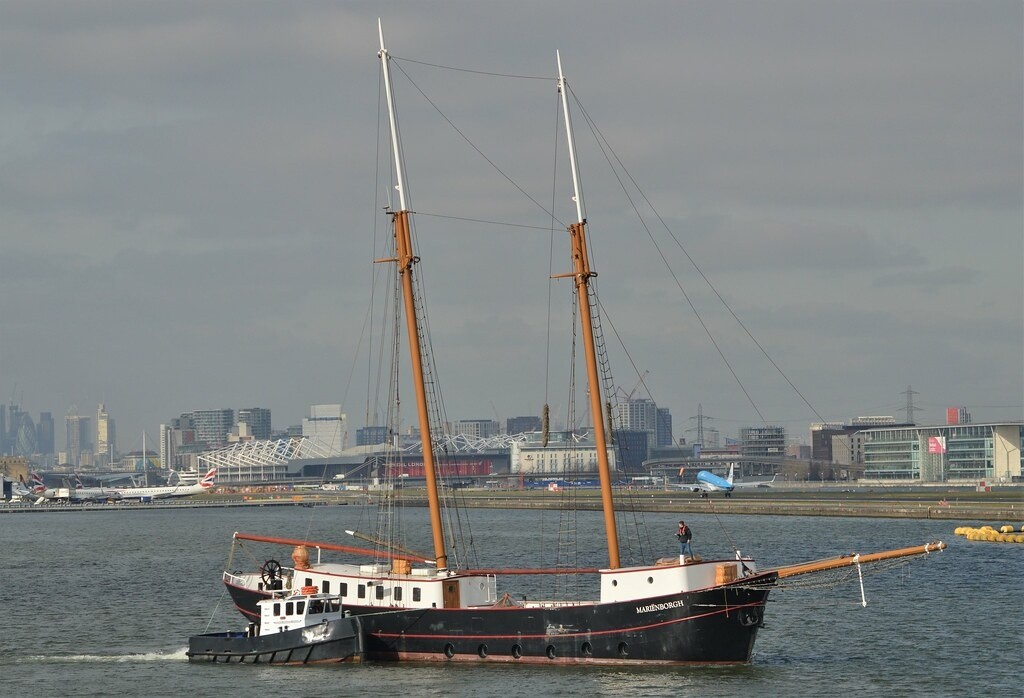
[301,586,318,594]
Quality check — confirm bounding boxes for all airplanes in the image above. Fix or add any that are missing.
[674,463,737,501]
[11,465,218,504]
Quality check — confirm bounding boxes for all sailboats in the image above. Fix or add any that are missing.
[222,18,952,672]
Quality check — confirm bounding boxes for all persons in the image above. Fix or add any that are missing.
[674,520,693,561]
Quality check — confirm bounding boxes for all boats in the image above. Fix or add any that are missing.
[184,587,365,676]
